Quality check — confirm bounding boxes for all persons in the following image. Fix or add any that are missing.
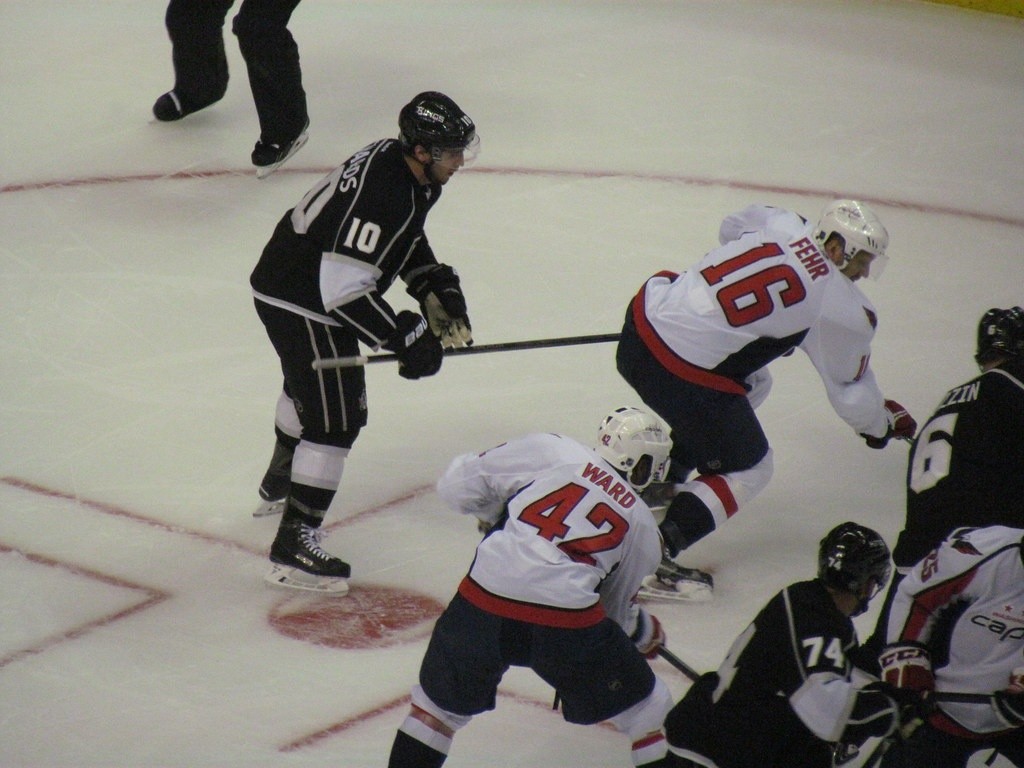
[249,90,480,594]
[616,197,918,599]
[877,523,1024,768]
[385,403,678,767]
[659,523,924,768]
[859,305,1024,679]
[153,0,309,179]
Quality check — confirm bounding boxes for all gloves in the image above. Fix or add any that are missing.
[632,608,665,659]
[858,399,917,449]
[383,310,443,380]
[865,681,927,744]
[880,644,934,702]
[407,264,473,350]
[990,666,1024,729]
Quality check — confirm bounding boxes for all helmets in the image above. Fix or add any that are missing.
[812,200,889,270]
[974,306,1024,365]
[399,92,475,153]
[818,522,892,591]
[597,406,672,490]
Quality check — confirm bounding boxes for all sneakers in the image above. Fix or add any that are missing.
[154,87,207,122]
[636,525,714,604]
[266,518,350,593]
[252,439,295,516]
[252,116,310,180]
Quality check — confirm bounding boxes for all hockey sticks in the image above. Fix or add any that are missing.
[861,739,891,768]
[654,644,700,682]
[904,436,914,444]
[310,332,622,370]
[929,689,990,705]
